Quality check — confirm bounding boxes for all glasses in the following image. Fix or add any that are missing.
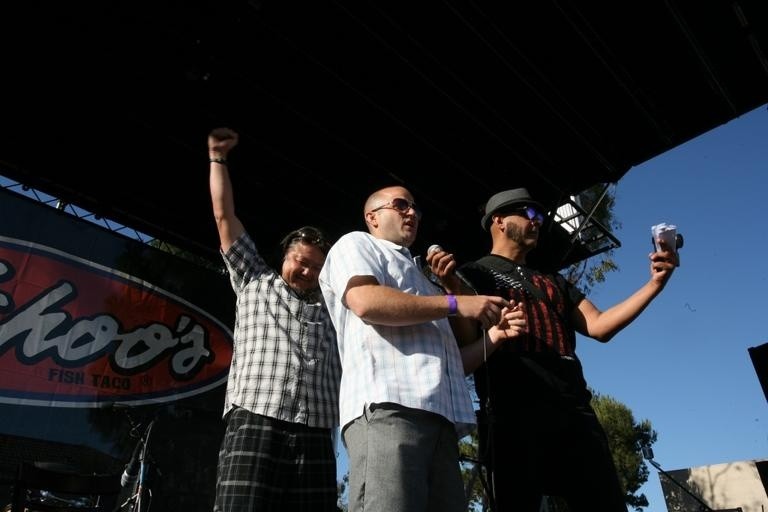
[294,228,331,254]
[500,205,544,227]
[370,197,421,215]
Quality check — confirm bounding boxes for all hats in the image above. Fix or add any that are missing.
[480,187,548,233]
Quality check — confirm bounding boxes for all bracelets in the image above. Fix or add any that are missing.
[446,294,458,316]
[209,158,228,165]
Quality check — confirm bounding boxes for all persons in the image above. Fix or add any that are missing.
[312,181,528,512]
[452,186,685,512]
[198,124,349,512]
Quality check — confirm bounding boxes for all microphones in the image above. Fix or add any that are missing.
[426,244,474,289]
[120,433,146,488]
[642,447,654,460]
